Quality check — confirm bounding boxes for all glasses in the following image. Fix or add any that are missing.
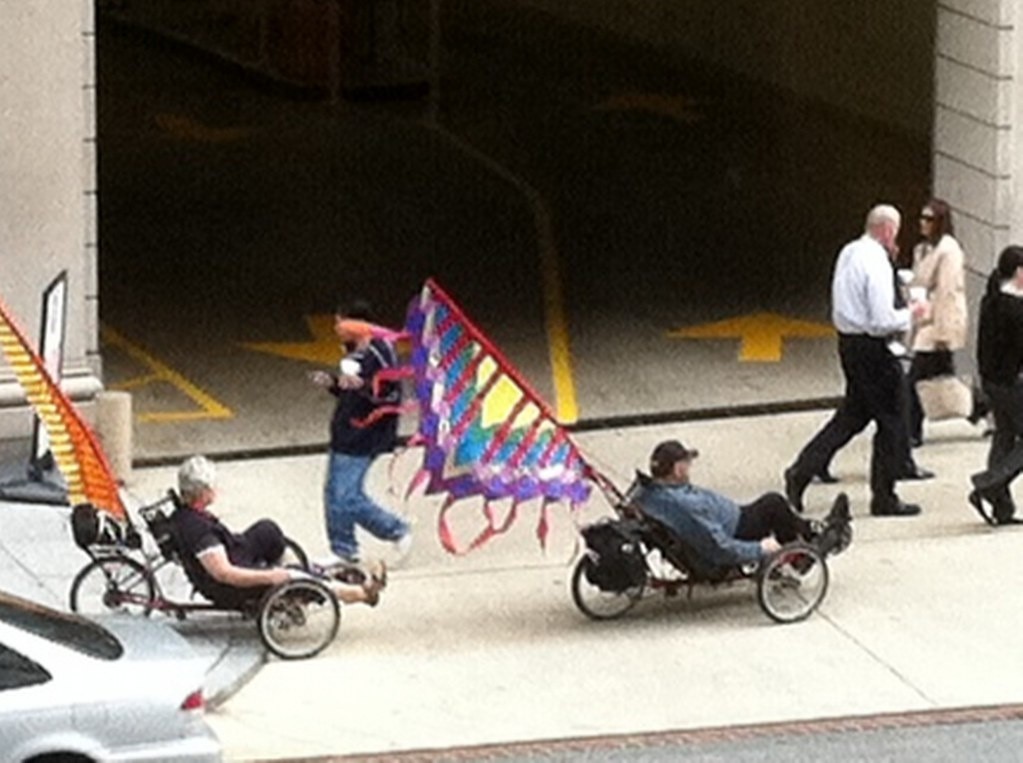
[919,213,935,221]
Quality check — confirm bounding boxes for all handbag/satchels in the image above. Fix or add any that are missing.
[914,343,973,420]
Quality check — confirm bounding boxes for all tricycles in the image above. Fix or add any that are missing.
[571,470,855,623]
[70,492,365,659]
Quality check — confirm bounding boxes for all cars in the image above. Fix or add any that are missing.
[1,592,221,762]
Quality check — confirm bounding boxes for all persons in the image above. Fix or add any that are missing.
[967,245,1023,528]
[165,455,386,609]
[901,199,994,447]
[308,302,415,570]
[650,441,849,575]
[784,209,934,517]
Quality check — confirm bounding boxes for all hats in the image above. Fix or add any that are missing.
[649,439,697,475]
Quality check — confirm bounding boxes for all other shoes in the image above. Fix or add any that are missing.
[786,463,810,512]
[817,467,836,483]
[875,498,921,516]
[903,468,934,479]
[1004,514,1022,525]
[969,491,998,527]
[396,519,416,558]
[796,528,841,571]
[823,494,849,535]
[363,561,387,605]
